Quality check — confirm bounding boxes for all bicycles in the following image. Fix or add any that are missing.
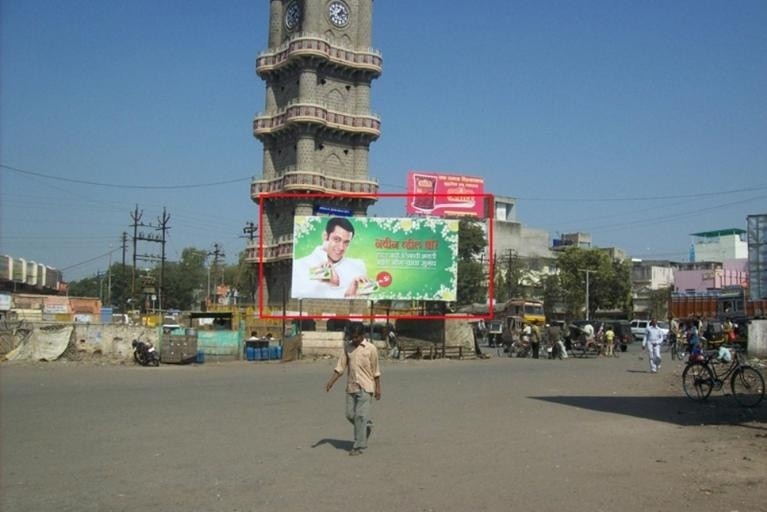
[682,344,765,408]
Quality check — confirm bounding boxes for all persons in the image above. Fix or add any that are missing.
[641,319,664,374]
[519,319,616,361]
[721,317,734,342]
[324,322,382,456]
[263,334,272,343]
[292,218,372,296]
[714,343,731,364]
[386,327,401,359]
[247,331,259,341]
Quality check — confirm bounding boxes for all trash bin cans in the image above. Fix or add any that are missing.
[162,325,198,364]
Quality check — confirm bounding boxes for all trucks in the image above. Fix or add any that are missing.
[460,298,545,338]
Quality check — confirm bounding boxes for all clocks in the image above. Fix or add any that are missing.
[284,1,301,30]
[324,0,351,29]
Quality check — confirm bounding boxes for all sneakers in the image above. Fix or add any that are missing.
[366,420,373,438]
[349,448,362,456]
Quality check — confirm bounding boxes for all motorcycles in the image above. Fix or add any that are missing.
[131,338,160,366]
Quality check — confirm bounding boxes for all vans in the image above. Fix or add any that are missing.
[629,320,669,342]
